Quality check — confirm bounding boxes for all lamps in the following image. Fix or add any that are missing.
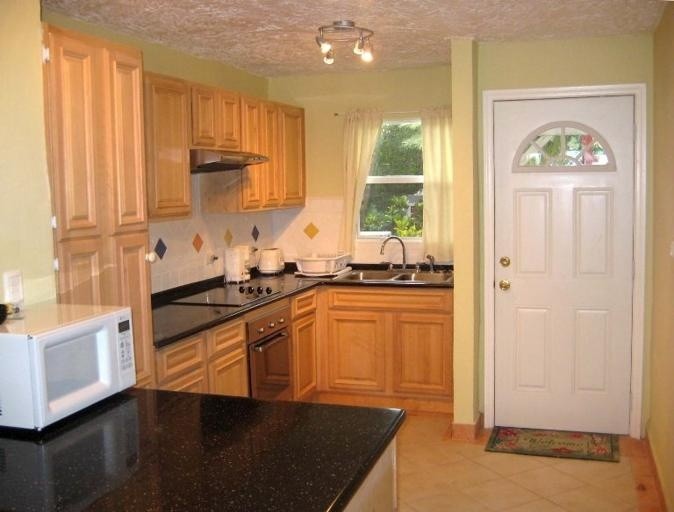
[316,21,376,66]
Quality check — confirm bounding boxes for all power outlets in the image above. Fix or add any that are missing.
[4,269,25,304]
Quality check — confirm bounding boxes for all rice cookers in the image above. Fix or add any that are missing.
[257,248,285,275]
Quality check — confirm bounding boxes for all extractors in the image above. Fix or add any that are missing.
[190,149,270,174]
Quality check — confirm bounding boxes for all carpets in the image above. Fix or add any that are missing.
[485,427,619,463]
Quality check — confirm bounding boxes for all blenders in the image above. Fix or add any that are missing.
[223,245,252,285]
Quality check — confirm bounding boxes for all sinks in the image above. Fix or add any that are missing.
[332,269,402,285]
[392,268,454,287]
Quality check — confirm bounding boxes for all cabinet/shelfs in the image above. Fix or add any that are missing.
[283,104,305,206]
[220,94,240,148]
[43,25,156,392]
[192,85,217,146]
[208,318,249,396]
[290,286,323,404]
[144,68,191,216]
[159,340,207,395]
[264,103,281,207]
[325,285,452,415]
[0,387,408,512]
[241,96,264,209]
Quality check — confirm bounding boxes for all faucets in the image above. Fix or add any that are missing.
[380,236,407,270]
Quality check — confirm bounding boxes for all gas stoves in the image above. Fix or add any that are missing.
[167,286,280,307]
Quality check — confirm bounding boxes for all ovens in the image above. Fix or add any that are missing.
[245,296,292,400]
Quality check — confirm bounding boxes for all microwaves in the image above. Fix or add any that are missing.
[0,303,137,430]
